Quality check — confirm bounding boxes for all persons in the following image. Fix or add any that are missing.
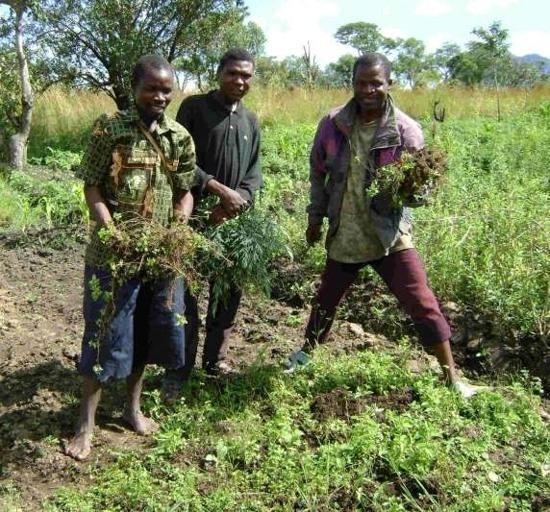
[176,46,264,379]
[65,54,196,460]
[291,54,472,398]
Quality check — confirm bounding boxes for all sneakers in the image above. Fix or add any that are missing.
[280,350,311,372]
[448,381,471,399]
[201,359,240,380]
